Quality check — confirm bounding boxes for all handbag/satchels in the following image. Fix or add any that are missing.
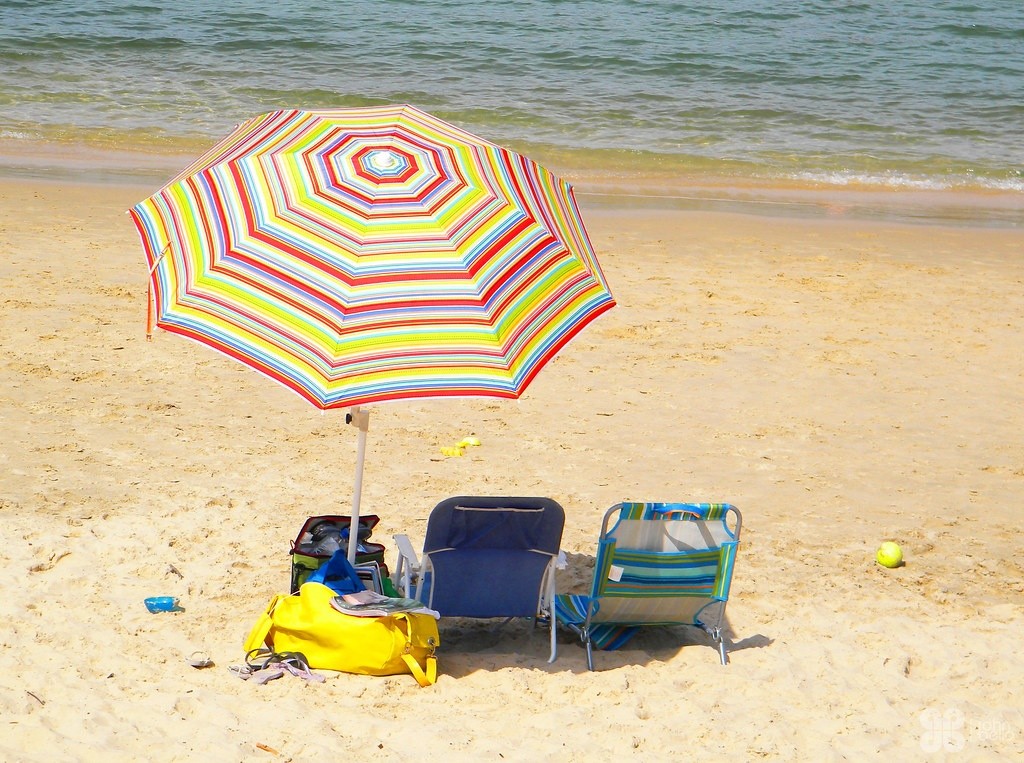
[244,582,440,687]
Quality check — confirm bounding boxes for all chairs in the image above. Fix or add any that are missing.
[546,501,743,671]
[391,498,567,664]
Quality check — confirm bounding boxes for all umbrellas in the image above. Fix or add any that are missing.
[124,102,620,564]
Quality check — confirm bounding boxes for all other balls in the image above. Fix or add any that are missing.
[877,540,904,568]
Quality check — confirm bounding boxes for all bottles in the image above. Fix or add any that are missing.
[308,528,348,554]
[145,597,180,614]
